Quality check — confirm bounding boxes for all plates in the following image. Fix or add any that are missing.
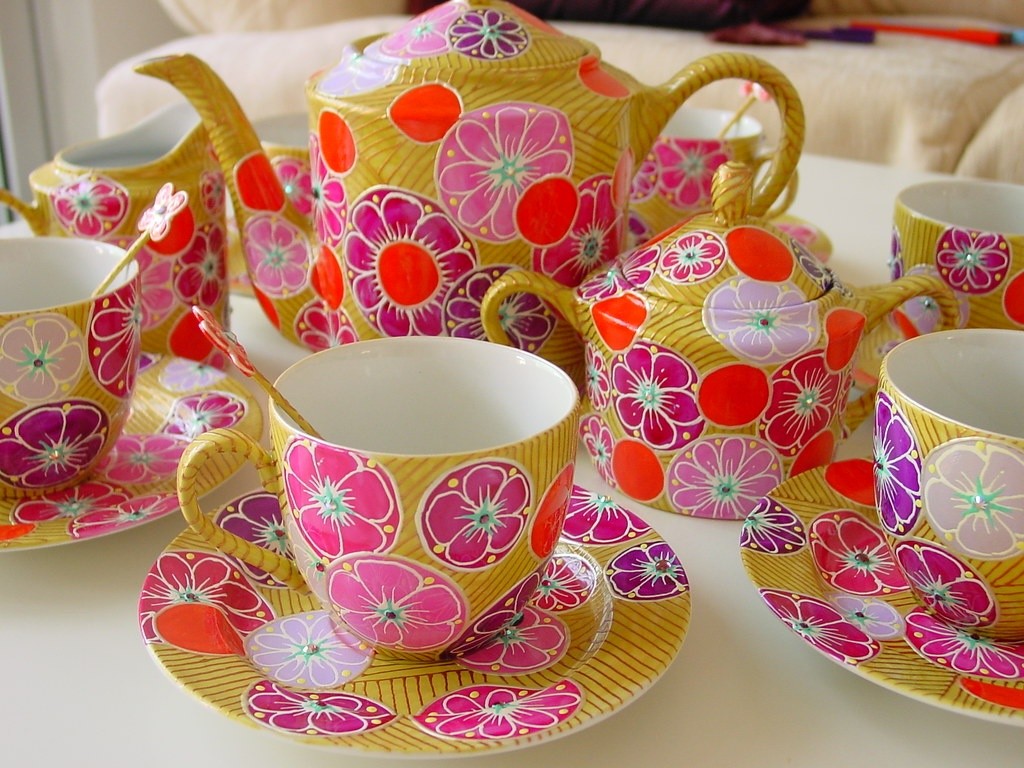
[0,353,262,554]
[760,209,834,263]
[739,457,1024,727]
[140,484,693,759]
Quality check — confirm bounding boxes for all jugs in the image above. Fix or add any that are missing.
[0,97,230,373]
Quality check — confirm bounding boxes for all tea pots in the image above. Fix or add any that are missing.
[479,162,960,520]
[135,0,806,401]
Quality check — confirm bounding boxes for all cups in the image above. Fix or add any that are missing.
[0,236,142,499]
[230,109,311,296]
[626,109,798,252]
[872,329,1024,637]
[890,178,1024,340]
[177,335,581,661]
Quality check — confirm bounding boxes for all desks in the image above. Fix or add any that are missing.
[0,149,1023,767]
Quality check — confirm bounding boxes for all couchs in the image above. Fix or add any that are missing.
[97,0,1023,187]
